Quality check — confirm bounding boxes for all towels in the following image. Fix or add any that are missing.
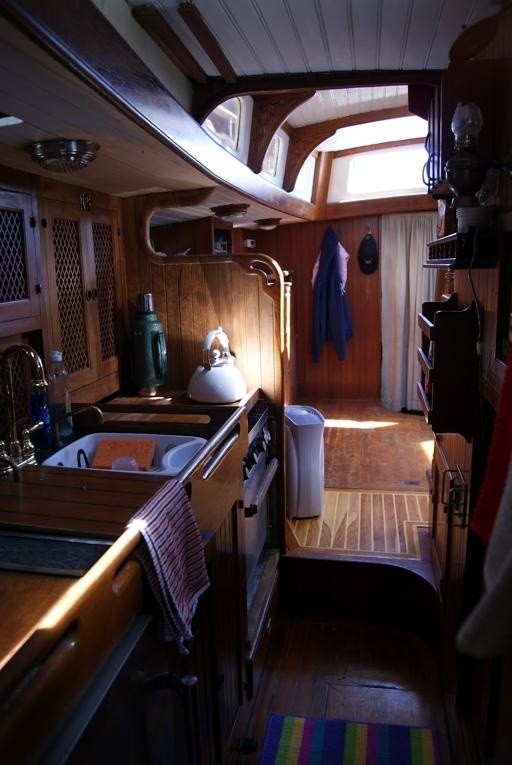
[124,478,212,656]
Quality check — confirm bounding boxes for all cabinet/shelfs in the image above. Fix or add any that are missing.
[0,189,42,338]
[422,58,512,272]
[40,197,128,396]
[417,293,485,443]
[151,216,233,255]
[429,440,473,600]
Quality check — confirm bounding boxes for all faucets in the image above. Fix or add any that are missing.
[51,407,104,445]
[0,342,47,446]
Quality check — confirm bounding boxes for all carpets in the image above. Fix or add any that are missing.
[259,712,440,765]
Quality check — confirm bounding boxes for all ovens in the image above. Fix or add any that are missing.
[242,424,278,585]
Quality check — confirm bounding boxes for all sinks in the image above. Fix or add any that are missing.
[41,433,203,473]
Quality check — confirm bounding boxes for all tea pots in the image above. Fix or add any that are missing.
[187,327,247,404]
